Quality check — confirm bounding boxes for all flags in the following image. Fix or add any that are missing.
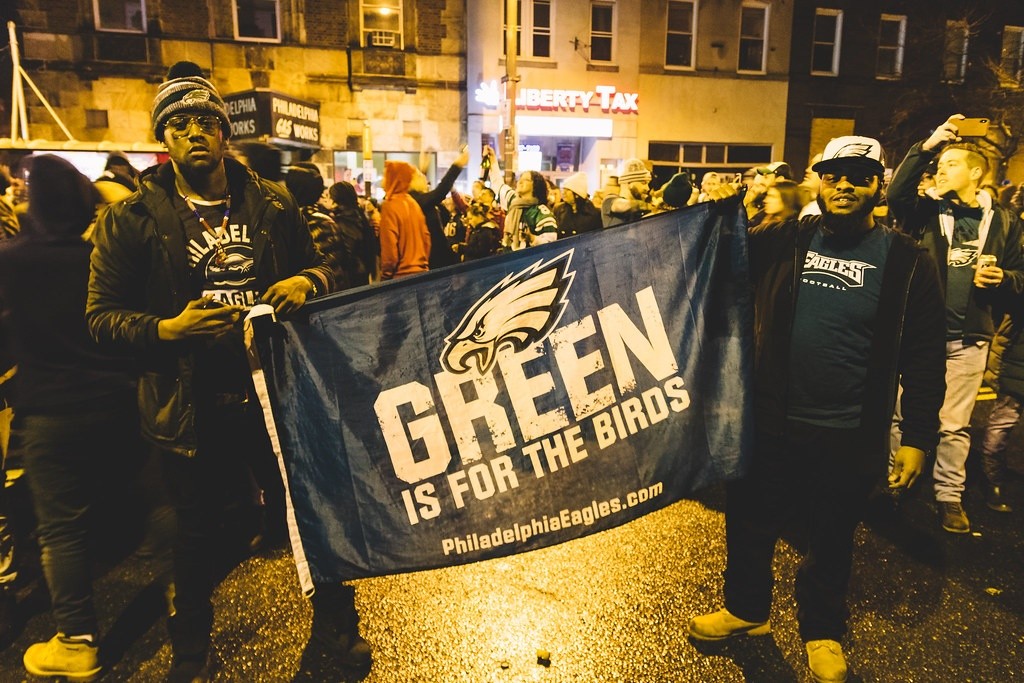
[232,187,759,599]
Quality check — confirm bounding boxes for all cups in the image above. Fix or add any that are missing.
[974,255,997,288]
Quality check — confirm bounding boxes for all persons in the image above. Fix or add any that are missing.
[687,135,949,683]
[0,155,114,678]
[0,113,1024,606]
[86,60,377,670]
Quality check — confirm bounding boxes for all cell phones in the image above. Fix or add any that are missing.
[203,299,229,309]
[948,118,990,137]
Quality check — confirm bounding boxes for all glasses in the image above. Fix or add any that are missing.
[162,115,224,139]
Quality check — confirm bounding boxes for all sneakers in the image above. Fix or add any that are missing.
[22,632,102,682]
[805,639,848,683]
[685,606,772,640]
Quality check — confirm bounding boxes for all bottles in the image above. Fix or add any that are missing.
[519,214,527,240]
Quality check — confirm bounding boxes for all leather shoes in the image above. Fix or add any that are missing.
[936,500,970,534]
[981,460,1014,513]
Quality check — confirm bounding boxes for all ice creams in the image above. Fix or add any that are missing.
[372,208,382,237]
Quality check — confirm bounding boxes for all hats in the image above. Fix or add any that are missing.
[757,161,792,180]
[618,159,652,185]
[811,135,886,180]
[561,170,589,199]
[662,172,692,206]
[150,61,233,144]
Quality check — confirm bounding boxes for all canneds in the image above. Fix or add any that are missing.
[973,254,997,289]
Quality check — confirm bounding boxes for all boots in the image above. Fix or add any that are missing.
[163,603,217,683]
[287,578,373,683]
